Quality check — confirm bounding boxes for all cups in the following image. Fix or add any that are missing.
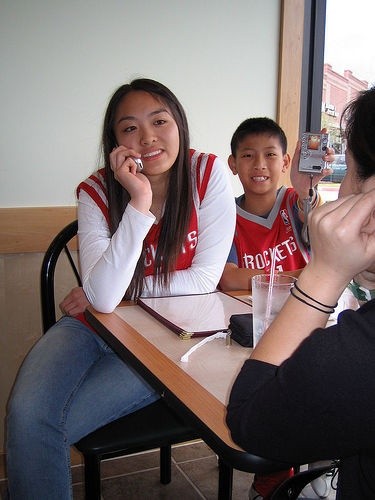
[251,274,298,349]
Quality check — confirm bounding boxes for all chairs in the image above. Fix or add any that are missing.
[40,218,200,500]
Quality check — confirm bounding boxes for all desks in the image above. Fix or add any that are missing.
[84,269,338,500]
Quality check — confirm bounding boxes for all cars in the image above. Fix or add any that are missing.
[321,154,347,183]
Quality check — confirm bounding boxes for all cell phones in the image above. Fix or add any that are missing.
[129,157,143,174]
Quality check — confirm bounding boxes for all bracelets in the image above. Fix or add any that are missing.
[290,280,338,314]
[266,270,278,288]
[353,282,375,301]
[295,189,323,214]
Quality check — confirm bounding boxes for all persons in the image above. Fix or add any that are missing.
[225,84,375,500]
[5,79,237,500]
[217,117,335,500]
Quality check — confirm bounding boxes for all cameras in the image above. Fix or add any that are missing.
[298,132,328,174]
